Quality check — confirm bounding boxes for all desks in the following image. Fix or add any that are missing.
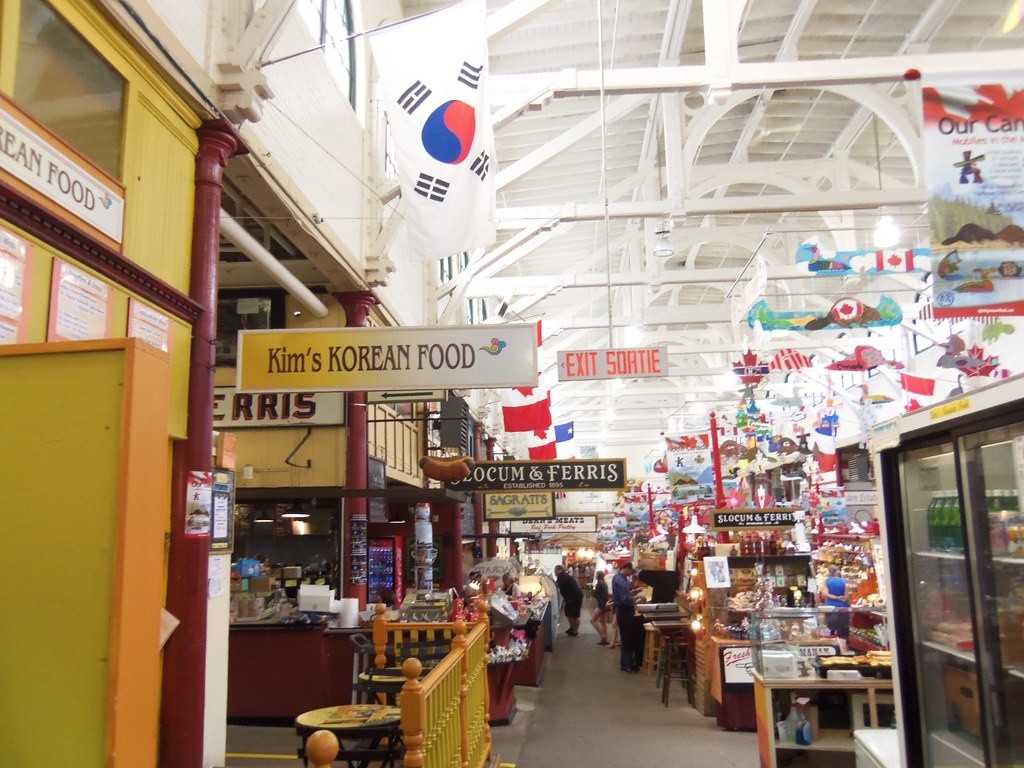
[358,666,434,768]
[296,704,402,768]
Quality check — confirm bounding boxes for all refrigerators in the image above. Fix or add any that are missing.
[871,374,1023,765]
[367,535,404,610]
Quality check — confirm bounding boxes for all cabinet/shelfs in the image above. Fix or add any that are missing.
[751,668,896,768]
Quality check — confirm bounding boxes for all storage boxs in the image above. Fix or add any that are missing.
[639,552,658,559]
[942,662,1024,751]
[638,559,660,570]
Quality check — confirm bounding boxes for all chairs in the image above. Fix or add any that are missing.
[349,667,433,768]
[396,638,453,668]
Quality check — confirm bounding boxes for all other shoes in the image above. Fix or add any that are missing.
[608,645,616,649]
[565,628,578,636]
[624,665,639,672]
[617,641,621,646]
[597,641,609,645]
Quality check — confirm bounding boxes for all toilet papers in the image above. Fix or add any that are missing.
[339,597,359,628]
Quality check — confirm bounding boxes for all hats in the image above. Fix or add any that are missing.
[624,561,639,572]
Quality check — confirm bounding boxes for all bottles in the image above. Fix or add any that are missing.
[728,620,801,641]
[740,531,776,555]
[383,561,393,572]
[927,489,964,553]
[370,561,382,572]
[985,490,1019,556]
[371,577,393,588]
[698,541,709,561]
[380,547,392,559]
[370,547,380,558]
[796,707,810,745]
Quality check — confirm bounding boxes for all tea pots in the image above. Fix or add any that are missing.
[854,510,880,535]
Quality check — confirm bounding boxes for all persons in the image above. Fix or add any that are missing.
[567,563,596,589]
[469,571,484,588]
[555,565,584,637]
[605,562,644,673]
[821,565,851,655]
[502,574,521,595]
[591,571,608,645]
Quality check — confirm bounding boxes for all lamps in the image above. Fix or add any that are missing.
[648,510,678,543]
[811,509,880,536]
[872,114,900,247]
[243,464,254,480]
[654,122,676,258]
[281,503,311,518]
[682,500,716,533]
[254,510,275,523]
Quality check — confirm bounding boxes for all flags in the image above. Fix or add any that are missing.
[499,319,557,462]
[666,433,709,451]
[624,493,646,506]
[553,421,574,443]
[369,0,498,264]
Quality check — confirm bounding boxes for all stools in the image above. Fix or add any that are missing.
[659,632,694,707]
[644,622,682,677]
